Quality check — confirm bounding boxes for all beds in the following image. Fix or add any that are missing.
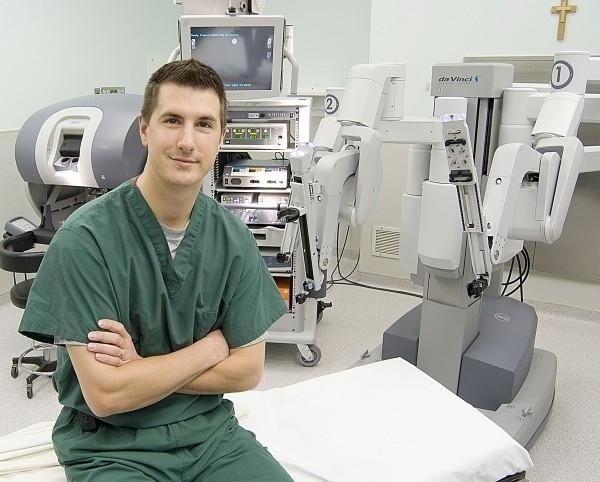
[1,354,532,481]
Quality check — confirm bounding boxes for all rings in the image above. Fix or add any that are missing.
[118,347,125,358]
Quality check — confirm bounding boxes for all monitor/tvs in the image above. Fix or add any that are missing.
[177,15,286,101]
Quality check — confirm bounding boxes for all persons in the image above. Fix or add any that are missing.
[17,56,294,480]
